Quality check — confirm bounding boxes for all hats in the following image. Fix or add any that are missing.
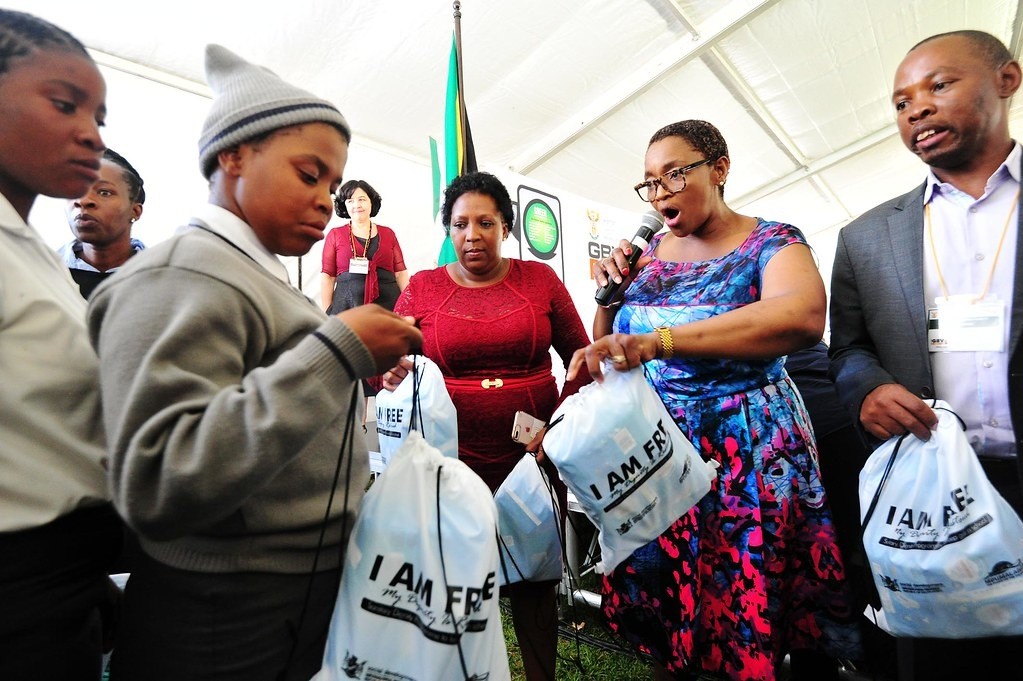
[198,42,352,181]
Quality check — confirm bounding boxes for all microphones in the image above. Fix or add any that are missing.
[594,210,666,306]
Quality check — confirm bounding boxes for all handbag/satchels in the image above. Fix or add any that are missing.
[494,451,563,597]
[375,351,459,477]
[542,355,721,576]
[858,383,1023,639]
[310,425,513,681]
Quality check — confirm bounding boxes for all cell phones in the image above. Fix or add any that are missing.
[511,410,546,445]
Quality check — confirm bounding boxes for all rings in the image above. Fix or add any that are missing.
[611,356,627,363]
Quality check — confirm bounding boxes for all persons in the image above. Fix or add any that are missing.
[0,9,114,681]
[381,172,594,681]
[85,45,423,680]
[55,149,147,302]
[784,337,900,681]
[567,120,825,680]
[826,31,1023,681]
[321,180,409,317]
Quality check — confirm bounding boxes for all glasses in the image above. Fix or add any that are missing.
[635,153,730,202]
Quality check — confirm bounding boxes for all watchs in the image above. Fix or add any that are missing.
[654,326,674,361]
